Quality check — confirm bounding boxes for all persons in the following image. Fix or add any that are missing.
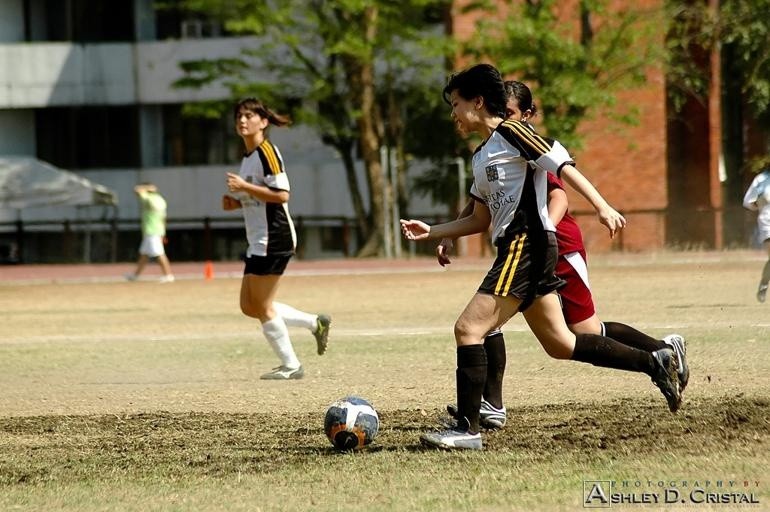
[435,81,691,430]
[401,63,683,451]
[743,160,770,304]
[222,95,331,383]
[123,182,175,284]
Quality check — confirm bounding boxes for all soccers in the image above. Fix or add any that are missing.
[325,397,378,447]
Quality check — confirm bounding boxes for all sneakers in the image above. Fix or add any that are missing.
[651,334,691,412]
[312,315,330,356]
[447,400,506,428]
[420,427,482,450]
[758,281,768,302]
[261,365,304,380]
